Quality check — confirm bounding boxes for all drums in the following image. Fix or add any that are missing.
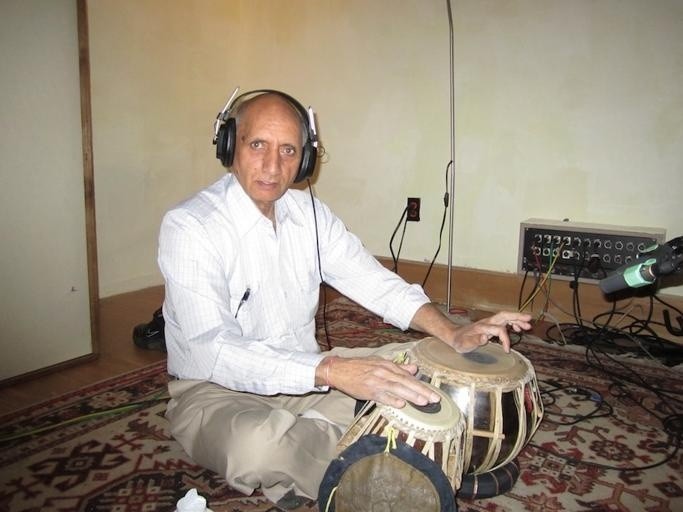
[335,379,467,499]
[403,337,544,478]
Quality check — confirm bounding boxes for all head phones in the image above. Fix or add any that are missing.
[212,84,319,184]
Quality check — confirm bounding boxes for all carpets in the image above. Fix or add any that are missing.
[1,296,682,510]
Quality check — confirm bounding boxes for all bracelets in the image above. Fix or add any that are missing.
[325,354,340,389]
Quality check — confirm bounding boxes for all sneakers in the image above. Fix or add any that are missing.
[132,306,167,353]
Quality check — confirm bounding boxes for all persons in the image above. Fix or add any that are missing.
[157,95,533,503]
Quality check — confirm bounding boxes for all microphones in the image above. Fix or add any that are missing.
[598,235,683,293]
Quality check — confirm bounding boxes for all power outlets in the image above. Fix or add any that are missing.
[407,198,420,221]
